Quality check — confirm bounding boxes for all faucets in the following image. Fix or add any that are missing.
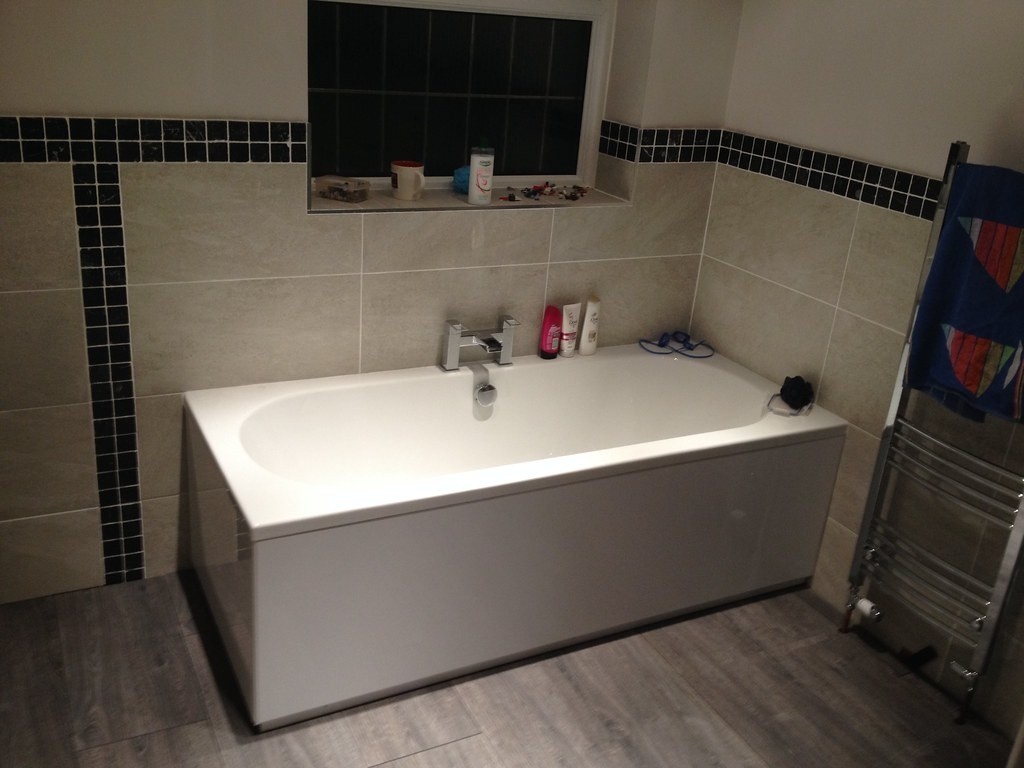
[441,314,522,371]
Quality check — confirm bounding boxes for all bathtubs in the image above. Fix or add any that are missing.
[177,335,848,735]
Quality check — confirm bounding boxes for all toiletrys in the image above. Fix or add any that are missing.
[468,146,495,204]
[579,296,600,357]
[538,305,561,360]
[559,302,582,358]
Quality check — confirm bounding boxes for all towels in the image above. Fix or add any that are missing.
[900,160,1024,424]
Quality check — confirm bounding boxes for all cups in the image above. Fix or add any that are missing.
[392,159,426,200]
